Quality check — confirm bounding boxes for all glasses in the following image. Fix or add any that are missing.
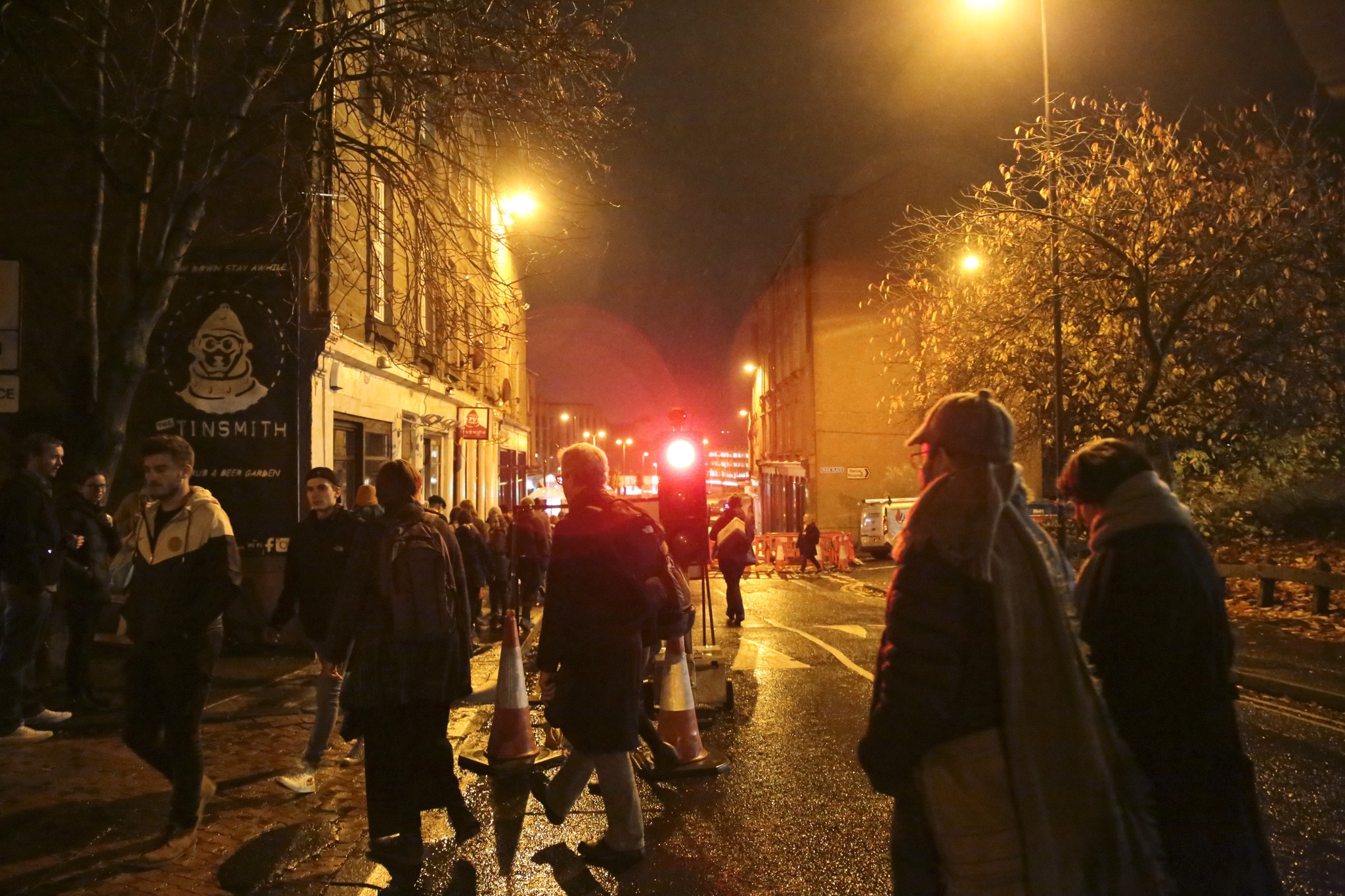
[556,472,571,485]
[908,448,932,468]
[83,483,109,490]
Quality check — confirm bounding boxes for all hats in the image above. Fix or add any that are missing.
[728,494,743,507]
[354,485,378,507]
[907,388,1016,461]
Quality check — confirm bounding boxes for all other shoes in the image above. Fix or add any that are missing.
[140,828,198,864]
[366,836,425,868]
[67,691,109,710]
[195,775,215,830]
[816,568,823,575]
[726,613,745,623]
[796,566,805,574]
[577,837,646,868]
[530,770,566,828]
[447,808,482,845]
[648,741,679,780]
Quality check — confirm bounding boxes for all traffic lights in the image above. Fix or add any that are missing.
[653,430,710,566]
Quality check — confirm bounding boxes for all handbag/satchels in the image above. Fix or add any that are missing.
[912,730,1029,896]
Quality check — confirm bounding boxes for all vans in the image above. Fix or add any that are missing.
[857,497,921,562]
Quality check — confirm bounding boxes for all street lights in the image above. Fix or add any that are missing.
[988,178,1069,557]
[583,427,606,447]
[614,438,633,495]
[560,412,576,445]
[639,451,649,473]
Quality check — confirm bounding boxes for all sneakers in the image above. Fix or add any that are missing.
[2,724,53,743]
[272,772,315,794]
[26,710,73,725]
[341,741,364,764]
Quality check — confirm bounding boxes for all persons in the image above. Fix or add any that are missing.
[0,431,86,747]
[795,513,823,575]
[302,485,385,767]
[426,494,566,633]
[322,458,484,866]
[529,442,661,865]
[709,493,756,626]
[57,466,116,712]
[1058,440,1288,896]
[857,389,1151,896]
[262,467,365,794]
[108,436,239,868]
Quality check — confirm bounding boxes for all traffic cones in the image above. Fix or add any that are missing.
[833,540,853,572]
[647,628,709,766]
[772,542,787,574]
[479,604,543,762]
[811,543,825,571]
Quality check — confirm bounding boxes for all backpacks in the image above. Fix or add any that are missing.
[812,526,821,544]
[586,498,696,642]
[367,509,459,644]
